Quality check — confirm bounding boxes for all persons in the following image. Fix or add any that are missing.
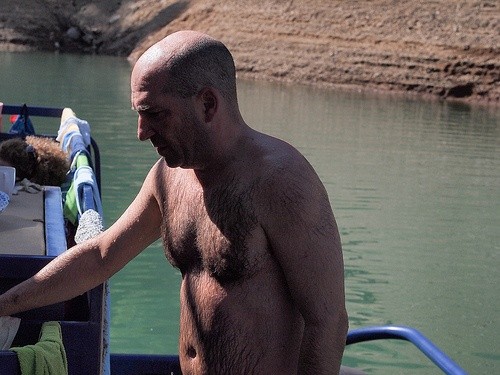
[1,31,348,375]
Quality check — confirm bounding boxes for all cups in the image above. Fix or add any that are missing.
[0,166,16,198]
